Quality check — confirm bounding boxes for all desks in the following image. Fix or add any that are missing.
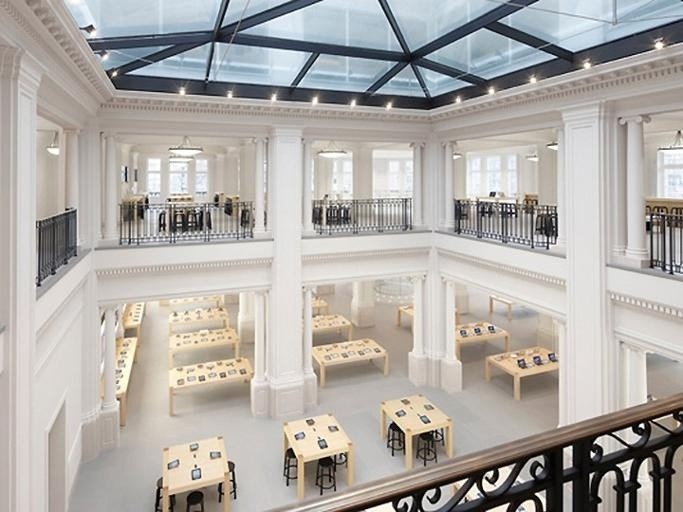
[282,414,354,500]
[161,434,230,512]
[100,295,390,427]
[380,394,453,471]
[396,295,560,400]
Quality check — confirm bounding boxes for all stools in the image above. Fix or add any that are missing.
[158,201,212,234]
[218,462,237,502]
[155,477,175,512]
[387,422,444,467]
[315,458,337,496]
[186,491,204,512]
[282,448,298,486]
